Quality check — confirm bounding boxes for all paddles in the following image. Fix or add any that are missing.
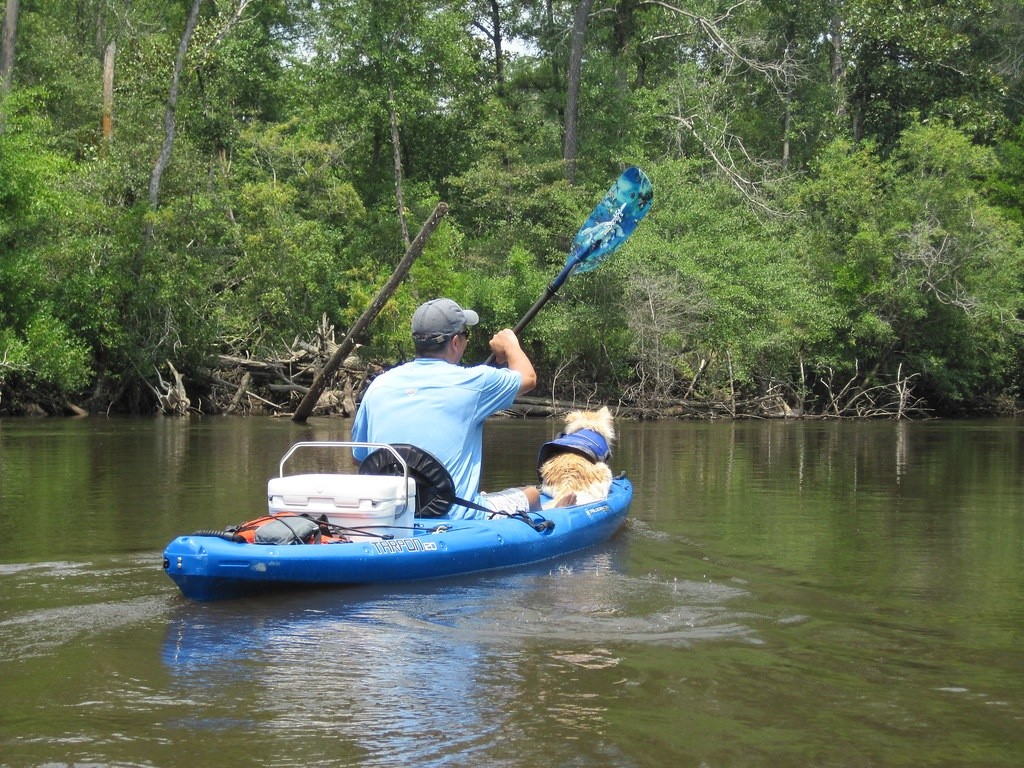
[486,165,654,367]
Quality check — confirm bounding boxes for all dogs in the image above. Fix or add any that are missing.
[537,405,616,510]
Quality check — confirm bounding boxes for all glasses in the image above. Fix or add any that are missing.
[451,328,470,341]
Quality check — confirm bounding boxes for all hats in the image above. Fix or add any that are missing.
[412,297,479,345]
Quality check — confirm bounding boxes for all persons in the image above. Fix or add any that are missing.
[351,298,579,521]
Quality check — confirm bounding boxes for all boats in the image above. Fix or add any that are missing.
[164,469,632,600]
